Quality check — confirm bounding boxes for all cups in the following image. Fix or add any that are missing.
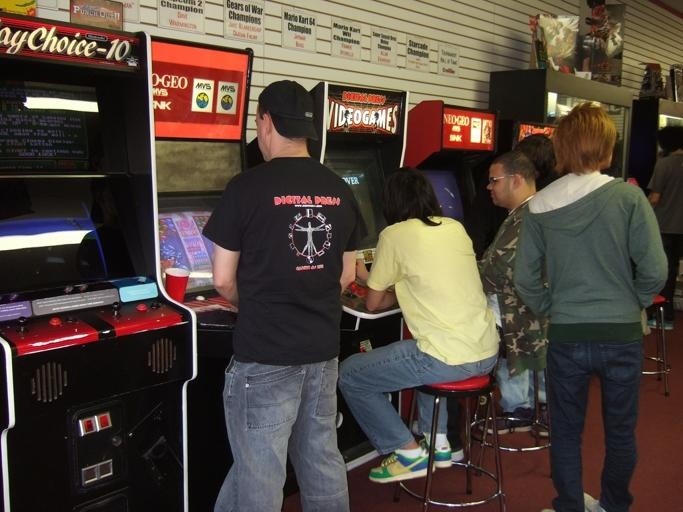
[164,267,189,304]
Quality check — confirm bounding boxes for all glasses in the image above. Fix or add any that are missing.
[488,174,516,184]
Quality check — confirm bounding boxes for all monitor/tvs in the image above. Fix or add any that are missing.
[325,156,388,254]
[154,192,221,295]
[1,177,112,311]
[421,164,476,235]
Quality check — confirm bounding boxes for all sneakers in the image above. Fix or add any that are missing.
[447,439,466,464]
[534,404,550,437]
[477,407,533,435]
[581,490,606,511]
[367,445,439,485]
[415,437,454,469]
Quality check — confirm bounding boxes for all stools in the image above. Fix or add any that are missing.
[388,338,555,511]
[642,293,671,401]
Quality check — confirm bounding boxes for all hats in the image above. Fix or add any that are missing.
[258,79,319,142]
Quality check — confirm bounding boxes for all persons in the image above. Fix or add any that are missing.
[199,80,369,511]
[513,130,561,193]
[645,124,682,331]
[511,99,671,512]
[336,167,499,486]
[477,150,550,436]
